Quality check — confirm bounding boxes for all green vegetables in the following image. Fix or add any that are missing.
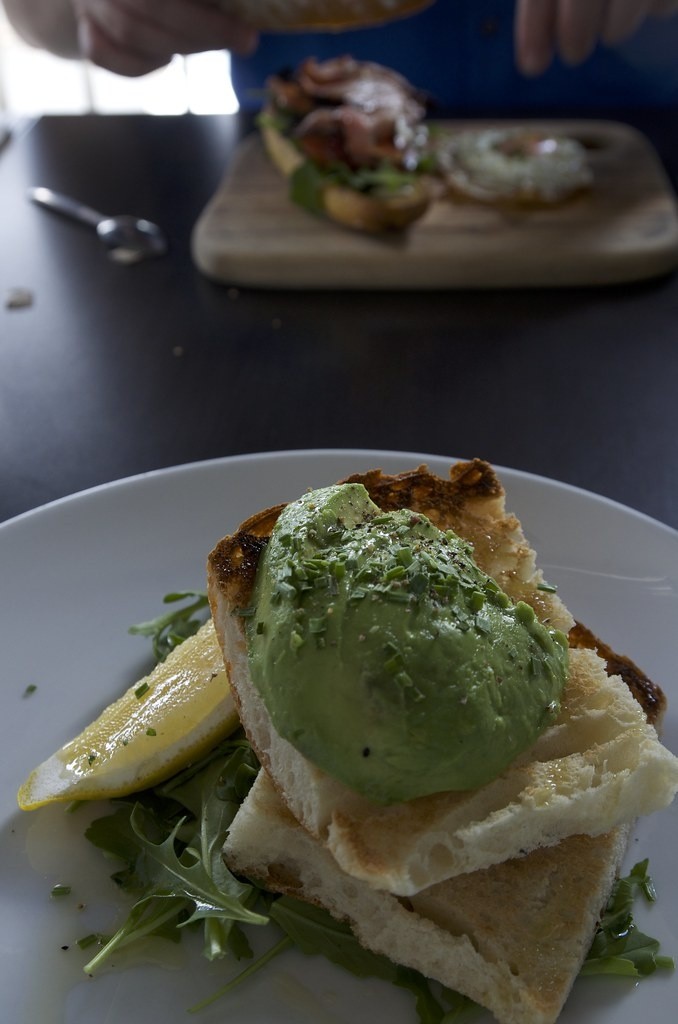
[72,589,673,1024]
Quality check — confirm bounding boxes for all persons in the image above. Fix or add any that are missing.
[0,0,677,113]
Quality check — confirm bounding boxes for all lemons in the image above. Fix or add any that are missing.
[18,617,240,812]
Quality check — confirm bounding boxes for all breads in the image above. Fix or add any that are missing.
[226,626,665,1024]
[207,461,678,894]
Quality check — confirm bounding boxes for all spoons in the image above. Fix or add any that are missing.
[31,186,164,265]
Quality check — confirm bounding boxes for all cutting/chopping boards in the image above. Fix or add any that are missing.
[192,120,678,286]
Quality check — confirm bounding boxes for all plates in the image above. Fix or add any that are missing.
[439,127,638,210]
[0,452,678,1024]
